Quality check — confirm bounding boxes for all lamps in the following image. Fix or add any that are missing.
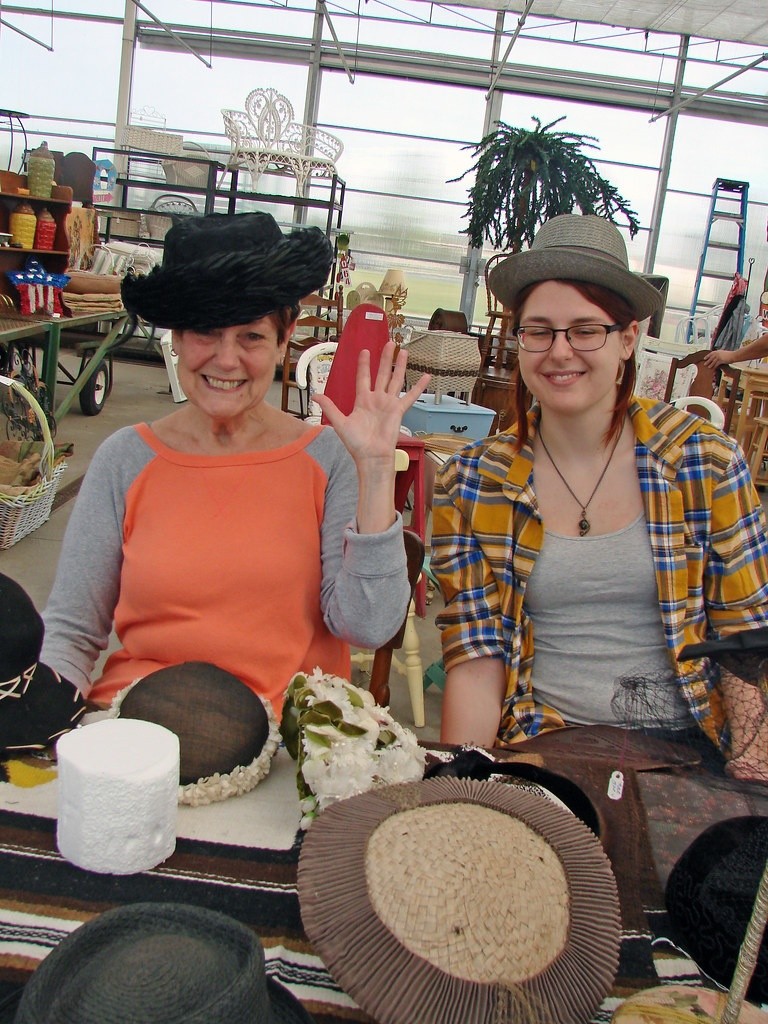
[379,270,405,316]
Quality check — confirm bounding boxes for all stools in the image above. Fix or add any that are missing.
[710,397,741,439]
[746,417,768,487]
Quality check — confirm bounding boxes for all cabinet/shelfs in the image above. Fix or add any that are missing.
[0,147,347,445]
[476,367,518,435]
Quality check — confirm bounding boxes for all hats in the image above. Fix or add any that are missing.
[487,213,663,323]
[0,571,87,760]
[120,211,335,330]
[657,815,768,1011]
[1,903,314,1024]
[108,659,281,805]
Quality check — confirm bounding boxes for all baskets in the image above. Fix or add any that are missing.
[99,209,142,238]
[144,194,197,242]
[120,125,184,156]
[0,376,67,550]
[163,140,211,188]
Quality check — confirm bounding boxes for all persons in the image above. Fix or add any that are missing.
[36,210,430,737]
[703,331,768,368]
[431,215,768,780]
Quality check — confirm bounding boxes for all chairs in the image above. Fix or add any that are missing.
[632,302,768,433]
[479,253,513,370]
[281,283,468,620]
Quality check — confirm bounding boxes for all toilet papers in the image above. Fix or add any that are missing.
[55,718,180,873]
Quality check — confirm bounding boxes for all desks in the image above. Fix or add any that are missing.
[720,362,768,443]
[0,693,768,1024]
[398,392,498,441]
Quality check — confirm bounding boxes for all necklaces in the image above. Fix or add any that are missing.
[537,414,626,536]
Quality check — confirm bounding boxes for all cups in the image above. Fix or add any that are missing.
[337,235,350,251]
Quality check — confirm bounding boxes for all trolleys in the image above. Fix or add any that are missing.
[0,169,141,416]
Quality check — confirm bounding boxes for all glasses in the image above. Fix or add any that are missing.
[511,323,624,352]
